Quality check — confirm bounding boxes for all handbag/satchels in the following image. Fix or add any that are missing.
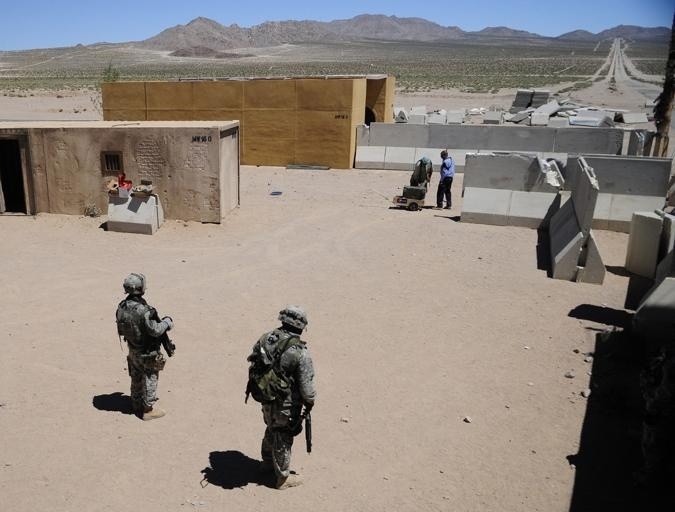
[410,175,418,186]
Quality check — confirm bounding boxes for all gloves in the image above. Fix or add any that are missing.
[301,403,313,416]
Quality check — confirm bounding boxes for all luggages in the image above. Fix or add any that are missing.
[403,185,426,200]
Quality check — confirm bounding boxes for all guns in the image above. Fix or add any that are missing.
[304,407,312,453]
[149,308,175,357]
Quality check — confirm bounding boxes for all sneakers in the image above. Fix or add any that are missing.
[264,473,276,488]
[432,206,442,210]
[136,402,157,417]
[444,205,451,209]
[140,408,166,420]
[276,472,303,489]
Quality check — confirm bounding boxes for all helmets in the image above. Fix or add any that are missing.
[123,272,146,294]
[278,306,308,329]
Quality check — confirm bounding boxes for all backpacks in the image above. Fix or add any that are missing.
[246,331,303,405]
[116,297,155,347]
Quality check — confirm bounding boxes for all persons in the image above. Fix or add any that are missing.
[115,272,174,423]
[409,156,434,193]
[249,301,316,491]
[431,150,455,210]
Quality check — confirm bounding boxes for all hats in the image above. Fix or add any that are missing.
[422,156,430,163]
